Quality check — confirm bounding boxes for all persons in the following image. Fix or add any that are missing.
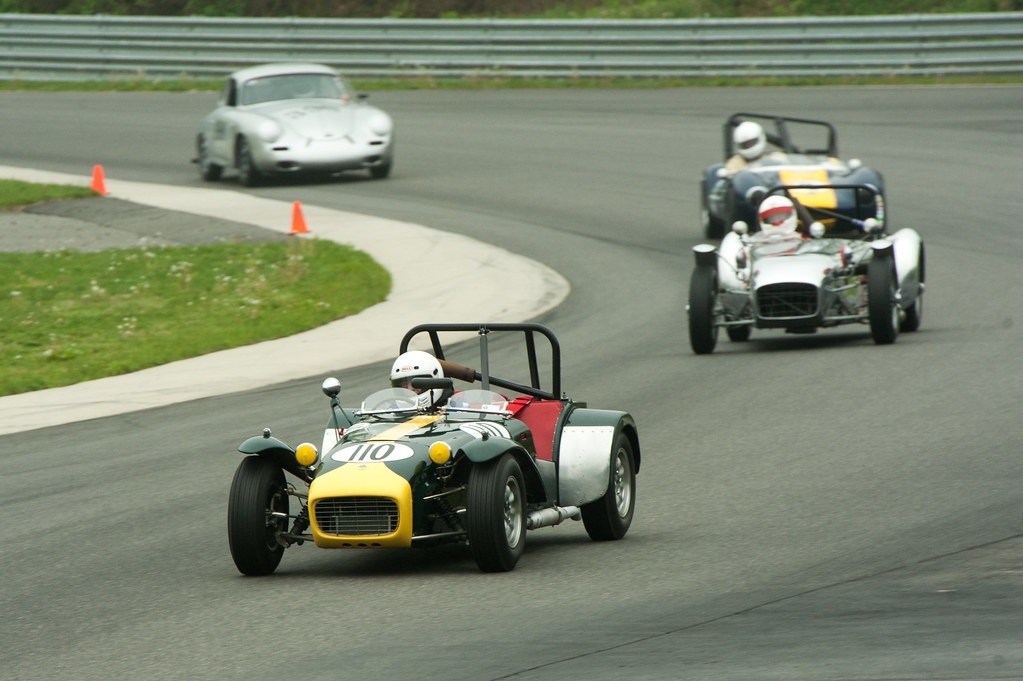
[726,122,767,168]
[391,351,451,406]
[759,195,799,232]
[289,77,319,97]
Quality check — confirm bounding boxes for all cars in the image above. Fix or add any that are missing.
[690,184,925,355]
[701,110,884,236]
[193,62,394,182]
[226,323,642,578]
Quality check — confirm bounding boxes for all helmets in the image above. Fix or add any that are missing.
[390,351,444,410]
[758,195,798,235]
[733,121,767,159]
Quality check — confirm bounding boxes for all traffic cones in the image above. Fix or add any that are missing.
[285,201,309,235]
[91,164,108,193]
